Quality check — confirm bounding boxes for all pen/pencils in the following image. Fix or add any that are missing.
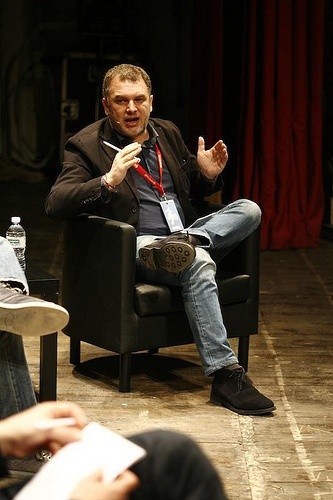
[37,417,75,430]
[103,140,140,162]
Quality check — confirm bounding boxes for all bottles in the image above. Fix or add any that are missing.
[6,217,26,273]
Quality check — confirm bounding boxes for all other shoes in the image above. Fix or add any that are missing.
[138,229,198,274]
[210,367,277,415]
[0,281,69,337]
[5,448,57,480]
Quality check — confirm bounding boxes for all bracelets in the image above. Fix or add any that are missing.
[102,173,116,191]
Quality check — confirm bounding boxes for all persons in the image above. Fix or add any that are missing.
[0,400,231,500]
[44,62,276,416]
[0,237,70,460]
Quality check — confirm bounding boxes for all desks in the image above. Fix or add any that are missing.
[24,260,60,404]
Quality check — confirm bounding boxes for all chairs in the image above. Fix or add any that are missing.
[59,133,259,393]
